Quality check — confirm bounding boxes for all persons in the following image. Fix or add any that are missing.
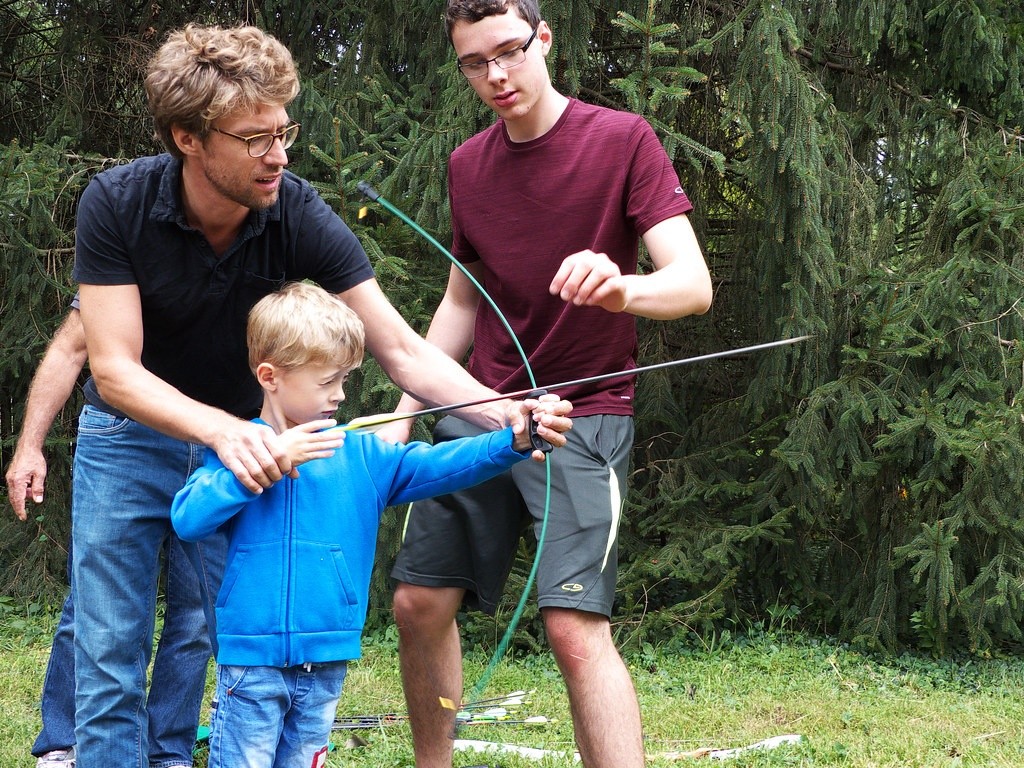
[71,26,573,768]
[350,0,713,768]
[172,282,559,768]
[6,290,90,768]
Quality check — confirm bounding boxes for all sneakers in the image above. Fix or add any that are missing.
[35,748,75,768]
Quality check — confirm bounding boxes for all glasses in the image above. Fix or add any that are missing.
[208,119,301,160]
[457,25,542,80]
